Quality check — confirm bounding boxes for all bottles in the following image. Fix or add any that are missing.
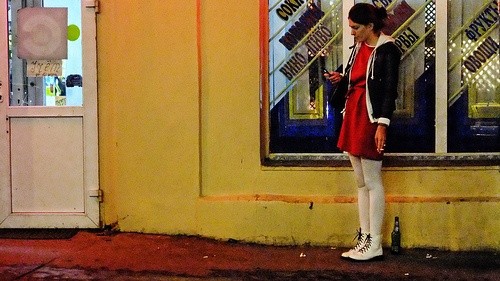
[391,216,401,254]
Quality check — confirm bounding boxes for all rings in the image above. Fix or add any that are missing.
[383,144,386,146]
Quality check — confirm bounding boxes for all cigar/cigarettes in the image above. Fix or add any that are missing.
[377,148,384,151]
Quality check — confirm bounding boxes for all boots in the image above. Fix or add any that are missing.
[348,233,384,262]
[341,228,369,260]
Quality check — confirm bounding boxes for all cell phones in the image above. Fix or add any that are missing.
[320,66,331,77]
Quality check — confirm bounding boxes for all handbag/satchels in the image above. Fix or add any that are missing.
[328,63,350,106]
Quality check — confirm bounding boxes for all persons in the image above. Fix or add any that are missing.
[323,3,404,262]
